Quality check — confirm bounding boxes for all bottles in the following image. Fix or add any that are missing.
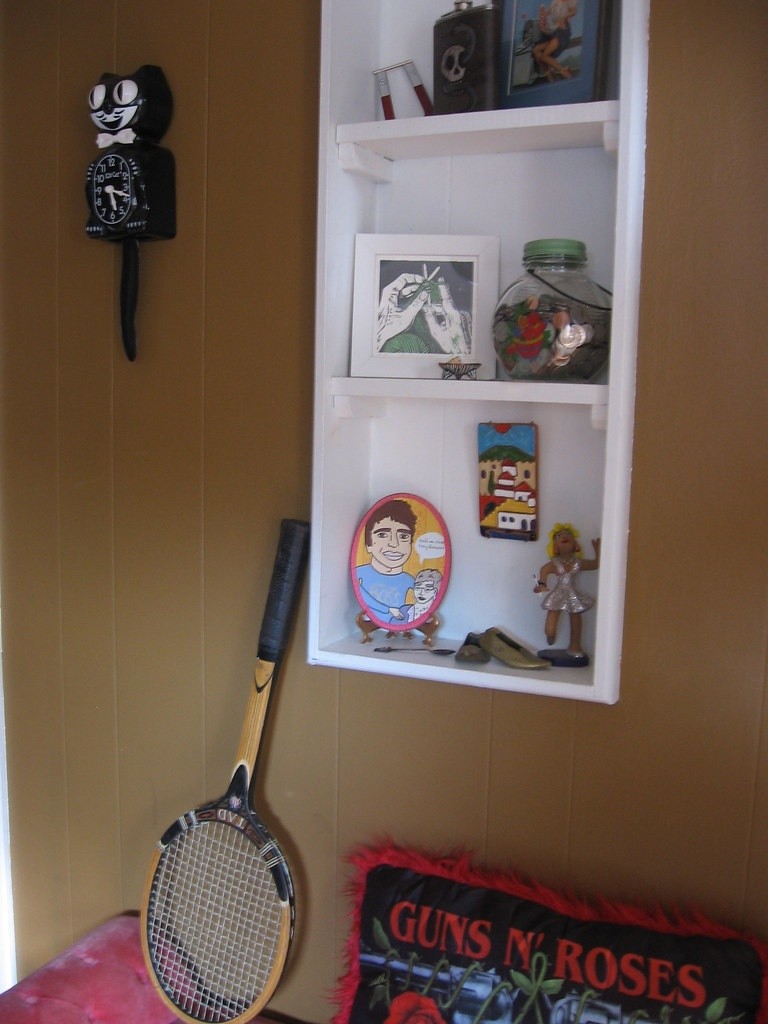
[493,240,609,383]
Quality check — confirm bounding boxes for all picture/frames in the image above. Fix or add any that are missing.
[487,0,611,111]
[350,232,498,381]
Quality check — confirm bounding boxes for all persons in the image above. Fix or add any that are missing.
[533,524,601,660]
[531,0,579,84]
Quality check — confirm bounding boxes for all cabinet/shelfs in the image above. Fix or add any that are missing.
[308,0,648,705]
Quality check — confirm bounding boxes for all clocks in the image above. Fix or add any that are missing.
[83,67,177,240]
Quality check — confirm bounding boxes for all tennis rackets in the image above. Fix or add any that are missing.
[139,517,311,1024]
